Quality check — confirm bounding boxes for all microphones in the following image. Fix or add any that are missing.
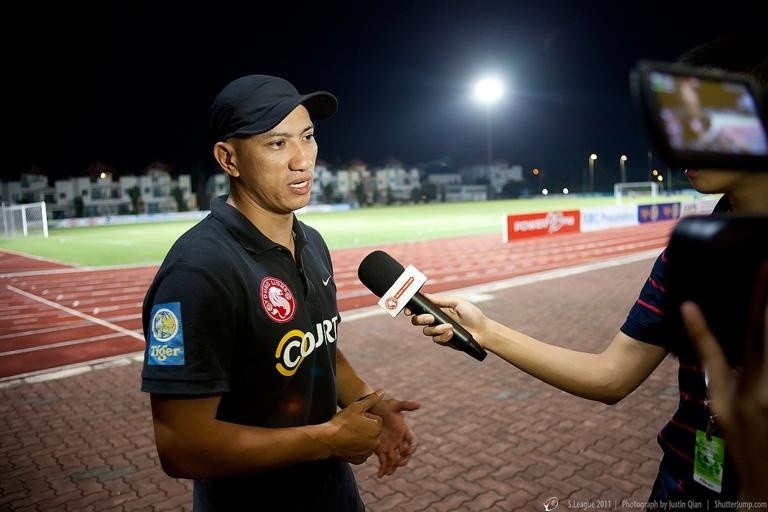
[357,251,488,361]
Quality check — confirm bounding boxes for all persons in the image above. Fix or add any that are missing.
[678,266,767,512]
[139,73,419,508]
[673,78,746,152]
[402,166,767,511]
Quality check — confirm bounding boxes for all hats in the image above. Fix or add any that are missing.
[208,76,339,141]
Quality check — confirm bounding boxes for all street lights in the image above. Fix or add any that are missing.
[587,153,598,192]
[468,73,511,201]
[618,153,629,181]
